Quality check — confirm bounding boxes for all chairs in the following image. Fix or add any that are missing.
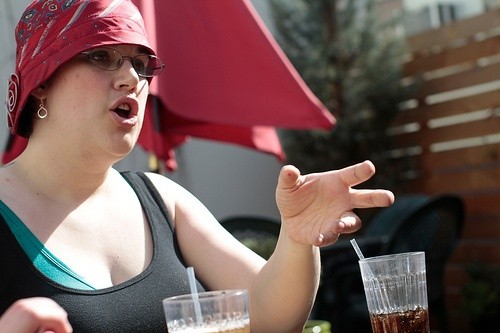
[309,191,467,333]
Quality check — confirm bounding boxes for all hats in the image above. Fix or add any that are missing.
[7,0,158,138]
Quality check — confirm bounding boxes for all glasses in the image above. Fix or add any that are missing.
[80,47,165,76]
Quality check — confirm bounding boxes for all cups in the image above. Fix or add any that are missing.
[163,289,250,333]
[359,252,430,333]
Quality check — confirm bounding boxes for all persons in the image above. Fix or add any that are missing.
[0,0,396,333]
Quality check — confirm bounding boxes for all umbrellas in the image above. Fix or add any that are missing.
[0,0,338,164]
